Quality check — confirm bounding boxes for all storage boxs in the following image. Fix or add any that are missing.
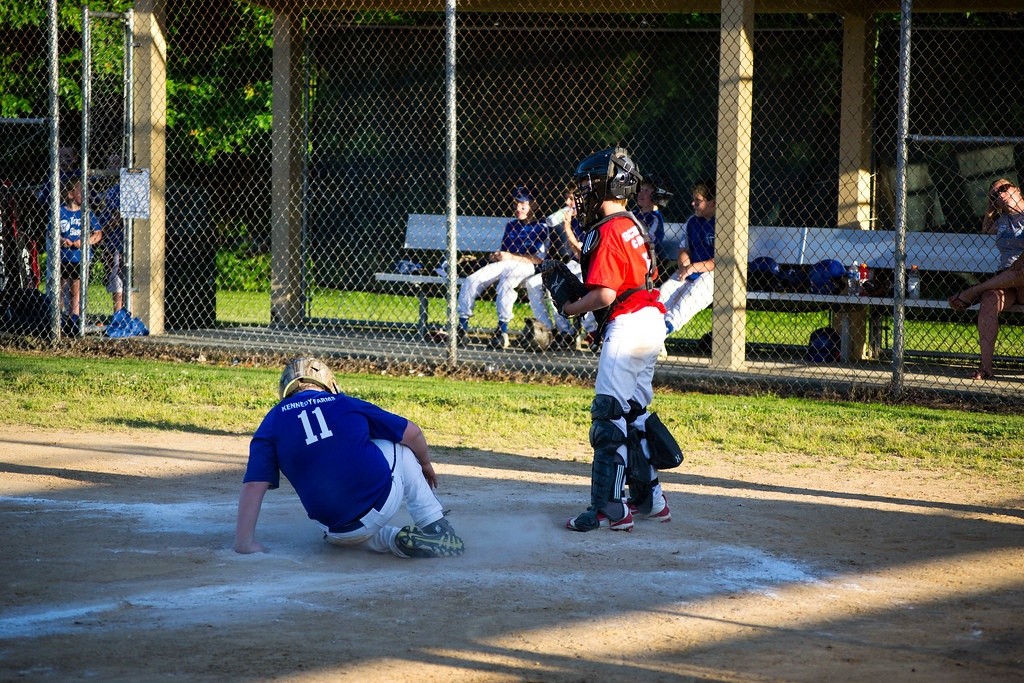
[833,310,868,360]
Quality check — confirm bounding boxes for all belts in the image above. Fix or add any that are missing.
[329,480,392,533]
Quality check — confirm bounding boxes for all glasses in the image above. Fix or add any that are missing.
[990,183,1015,202]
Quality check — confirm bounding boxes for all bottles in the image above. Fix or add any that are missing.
[859,263,869,296]
[908,265,920,300]
[848,261,859,296]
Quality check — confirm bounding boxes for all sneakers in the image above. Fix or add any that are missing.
[566,503,634,531]
[395,525,465,556]
[630,494,671,523]
[423,509,456,535]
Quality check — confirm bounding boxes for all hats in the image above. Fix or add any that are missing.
[510,186,538,202]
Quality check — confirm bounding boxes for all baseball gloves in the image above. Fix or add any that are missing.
[540,258,588,320]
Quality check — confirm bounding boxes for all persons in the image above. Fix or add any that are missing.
[546,169,672,355]
[948,178,1024,380]
[658,182,716,357]
[36,143,125,330]
[234,356,466,558]
[430,186,552,349]
[543,146,670,533]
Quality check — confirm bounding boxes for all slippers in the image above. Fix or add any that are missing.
[948,291,969,311]
[972,370,992,380]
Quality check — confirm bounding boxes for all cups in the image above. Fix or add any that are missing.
[545,206,575,227]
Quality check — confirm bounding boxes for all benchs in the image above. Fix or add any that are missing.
[373,213,1024,367]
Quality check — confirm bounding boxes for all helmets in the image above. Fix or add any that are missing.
[810,258,847,294]
[749,256,780,290]
[573,146,640,230]
[808,327,842,361]
[517,317,552,351]
[278,356,339,399]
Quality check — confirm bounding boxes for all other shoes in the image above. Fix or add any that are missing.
[455,332,469,346]
[489,333,510,348]
